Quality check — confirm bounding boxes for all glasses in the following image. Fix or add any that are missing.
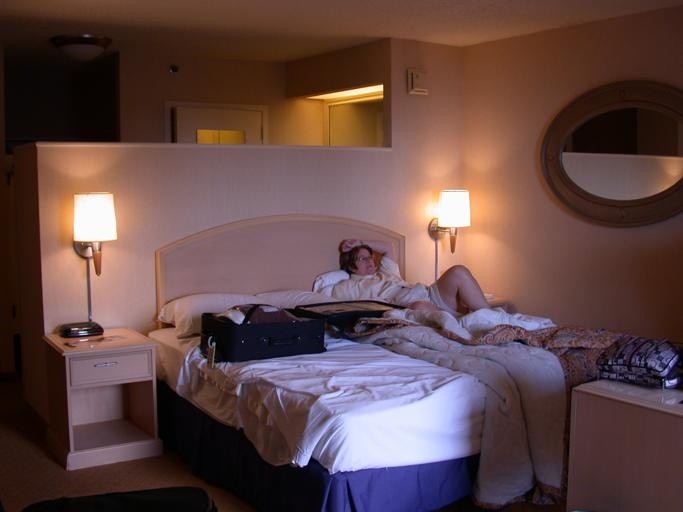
[359,256,374,263]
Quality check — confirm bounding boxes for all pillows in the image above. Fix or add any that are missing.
[153,269,353,337]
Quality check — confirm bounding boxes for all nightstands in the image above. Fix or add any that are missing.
[42,326,162,472]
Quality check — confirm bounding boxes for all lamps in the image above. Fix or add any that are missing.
[428,186,472,254]
[53,34,112,64]
[71,192,119,277]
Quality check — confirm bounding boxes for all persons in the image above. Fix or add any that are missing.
[332,238,490,312]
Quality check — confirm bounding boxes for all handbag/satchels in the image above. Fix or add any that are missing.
[596,334,681,388]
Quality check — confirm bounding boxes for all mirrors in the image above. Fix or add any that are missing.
[539,79,683,227]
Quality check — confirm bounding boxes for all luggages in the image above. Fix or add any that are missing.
[200,299,329,362]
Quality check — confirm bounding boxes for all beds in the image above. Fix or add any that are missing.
[151,212,631,511]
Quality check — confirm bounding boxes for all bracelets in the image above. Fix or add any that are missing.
[361,241,363,246]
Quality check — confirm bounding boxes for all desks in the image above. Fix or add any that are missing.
[565,380,683,511]
